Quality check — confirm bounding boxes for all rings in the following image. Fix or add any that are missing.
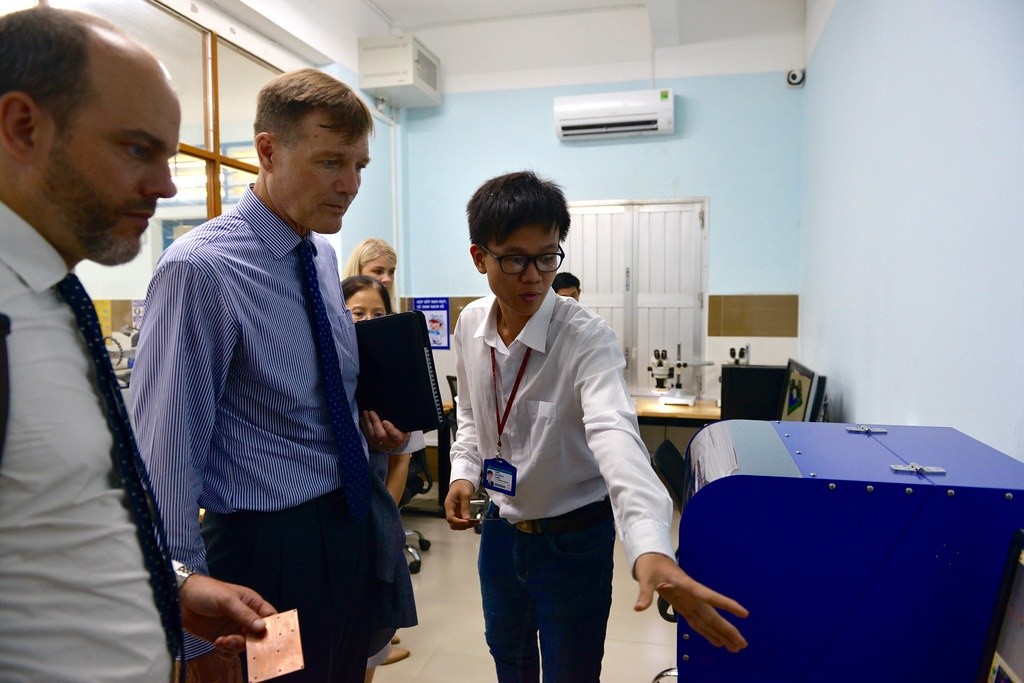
[376,440,384,448]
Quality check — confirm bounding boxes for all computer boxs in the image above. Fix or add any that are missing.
[720,364,788,422]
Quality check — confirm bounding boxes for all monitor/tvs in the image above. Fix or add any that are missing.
[780,358,826,422]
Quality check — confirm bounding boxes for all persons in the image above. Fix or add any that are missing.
[0,6,276,683]
[444,171,748,683]
[127,69,406,683]
[343,237,410,312]
[552,272,581,301]
[487,470,494,485]
[341,275,426,683]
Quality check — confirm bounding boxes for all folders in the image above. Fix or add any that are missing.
[354,309,447,431]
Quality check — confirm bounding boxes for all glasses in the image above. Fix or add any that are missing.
[478,243,565,275]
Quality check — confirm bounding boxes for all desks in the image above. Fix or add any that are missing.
[631,394,721,427]
[400,397,455,518]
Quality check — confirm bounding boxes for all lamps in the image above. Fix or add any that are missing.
[119,325,140,336]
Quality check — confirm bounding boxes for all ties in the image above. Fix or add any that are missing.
[56,270,184,683]
[291,238,376,524]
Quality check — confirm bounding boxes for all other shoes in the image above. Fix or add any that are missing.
[391,634,401,644]
[378,646,411,665]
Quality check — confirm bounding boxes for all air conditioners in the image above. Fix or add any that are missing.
[553,87,676,142]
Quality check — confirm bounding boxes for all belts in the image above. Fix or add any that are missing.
[496,505,612,533]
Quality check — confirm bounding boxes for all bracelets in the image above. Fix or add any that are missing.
[175,566,196,589]
